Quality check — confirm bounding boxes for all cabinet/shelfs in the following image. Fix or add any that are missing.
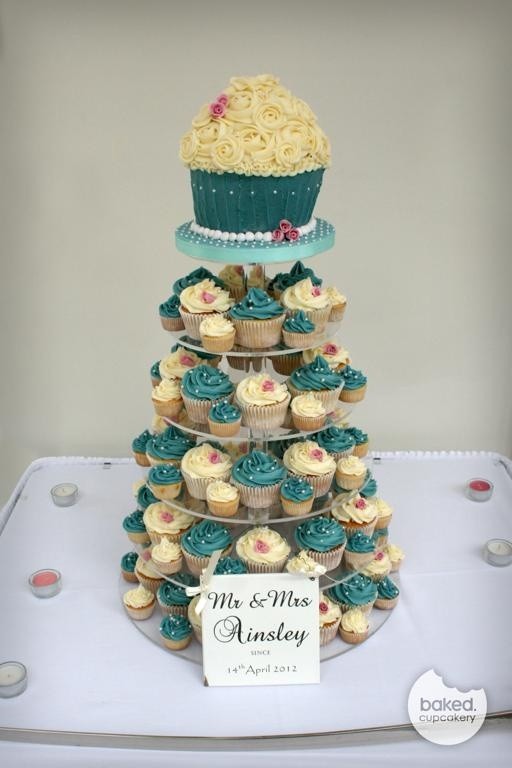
[117,216,399,666]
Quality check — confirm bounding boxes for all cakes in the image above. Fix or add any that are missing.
[330,485,378,537]
[382,543,406,573]
[179,363,236,425]
[150,535,184,574]
[179,439,234,501]
[145,424,196,468]
[159,613,195,652]
[151,377,185,419]
[281,305,316,350]
[206,399,243,438]
[344,427,369,459]
[134,543,162,595]
[340,607,372,644]
[135,483,161,510]
[201,552,246,575]
[335,453,368,490]
[283,439,337,499]
[158,292,188,333]
[143,461,182,501]
[361,551,392,578]
[172,267,227,298]
[283,548,327,578]
[325,571,378,615]
[316,425,355,460]
[131,427,151,468]
[122,580,156,621]
[229,447,288,510]
[286,353,346,415]
[358,468,379,499]
[177,70,332,243]
[229,285,287,349]
[336,365,368,404]
[143,500,196,546]
[187,594,203,647]
[217,262,272,298]
[316,589,343,646]
[155,572,200,618]
[178,510,234,581]
[158,346,206,378]
[312,341,350,373]
[233,525,292,573]
[198,314,237,355]
[343,528,377,565]
[149,360,162,389]
[368,495,393,530]
[120,551,140,585]
[293,513,348,571]
[170,334,224,370]
[290,389,328,432]
[206,479,240,517]
[322,284,348,323]
[176,276,235,343]
[281,275,333,334]
[269,259,323,303]
[235,371,293,430]
[132,479,143,496]
[374,525,389,546]
[374,576,399,611]
[122,508,149,544]
[277,474,316,516]
